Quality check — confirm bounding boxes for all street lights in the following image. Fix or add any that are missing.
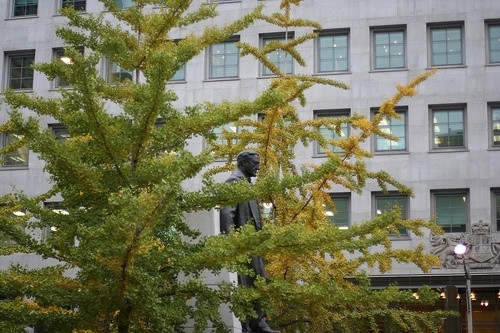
[454,243,473,332]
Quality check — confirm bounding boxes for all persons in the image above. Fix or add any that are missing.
[218,151,283,333]
